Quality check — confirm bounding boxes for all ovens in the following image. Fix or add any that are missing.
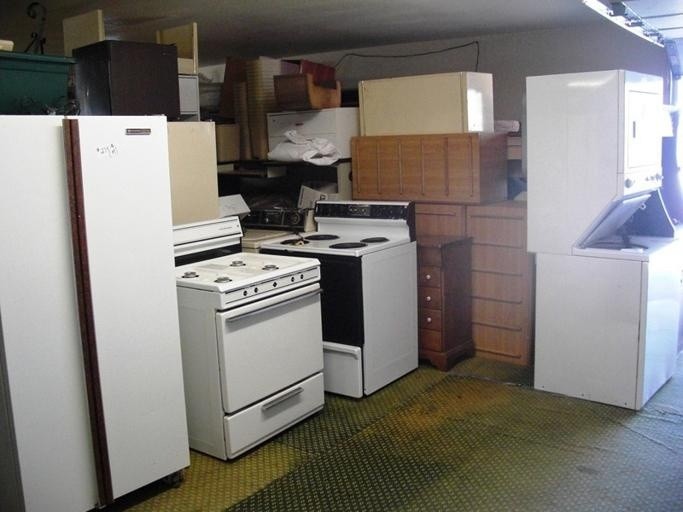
[316,241,419,403]
[175,283,323,463]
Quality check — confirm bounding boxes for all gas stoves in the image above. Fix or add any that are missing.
[260,230,411,258]
[177,252,321,312]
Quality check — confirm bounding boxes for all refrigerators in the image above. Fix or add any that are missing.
[1,114,189,512]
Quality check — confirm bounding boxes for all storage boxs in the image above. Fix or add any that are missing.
[0,49,77,114]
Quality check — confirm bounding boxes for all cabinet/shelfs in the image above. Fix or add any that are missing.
[358,72,494,137]
[413,203,467,236]
[268,107,357,159]
[351,131,508,205]
[63,10,219,227]
[414,233,473,370]
[273,60,341,108]
[467,201,530,367]
